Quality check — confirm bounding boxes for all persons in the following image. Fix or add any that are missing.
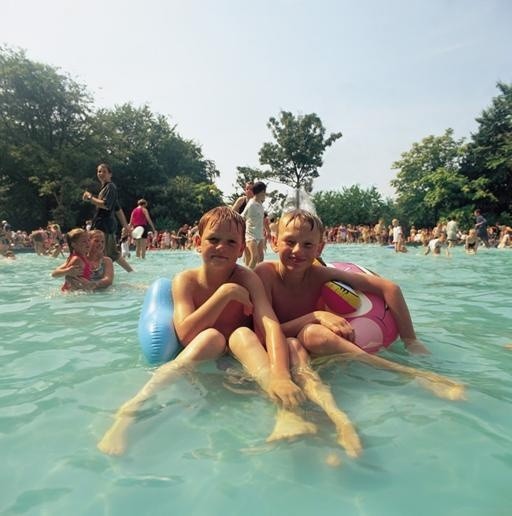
[82,163,134,273]
[0,180,280,292]
[324,209,512,257]
[253,209,467,459]
[97,206,317,456]
